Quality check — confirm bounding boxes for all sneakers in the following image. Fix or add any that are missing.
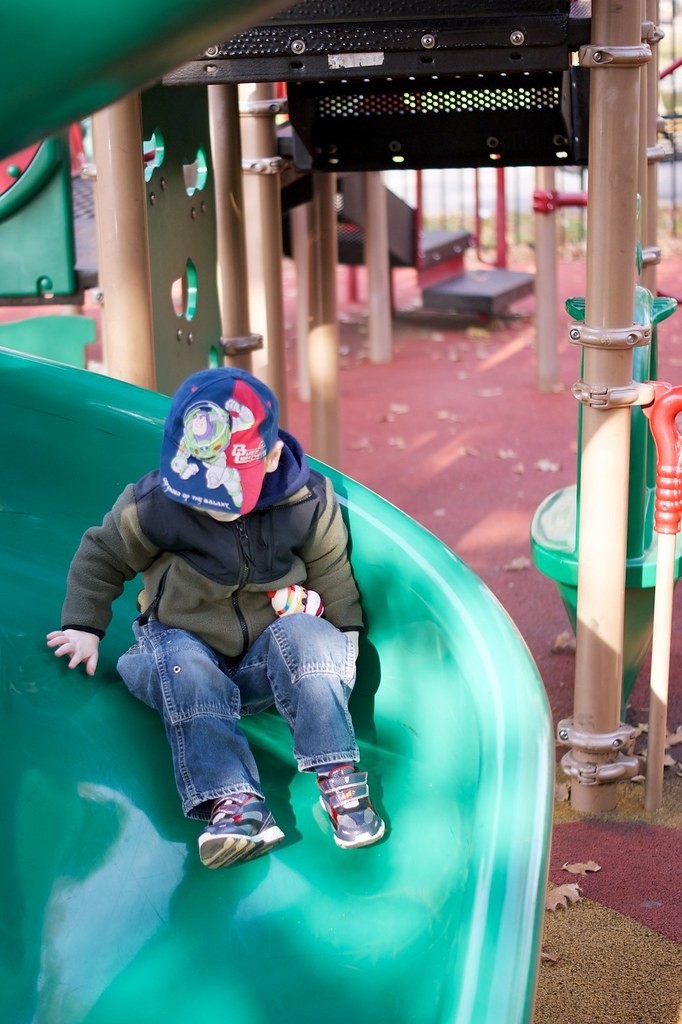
[198,792,285,870]
[317,766,386,849]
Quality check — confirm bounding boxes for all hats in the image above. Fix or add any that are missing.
[161,367,278,515]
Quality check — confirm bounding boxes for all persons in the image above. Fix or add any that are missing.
[44,363,387,872]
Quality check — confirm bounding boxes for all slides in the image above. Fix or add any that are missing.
[0,0,554,1024]
